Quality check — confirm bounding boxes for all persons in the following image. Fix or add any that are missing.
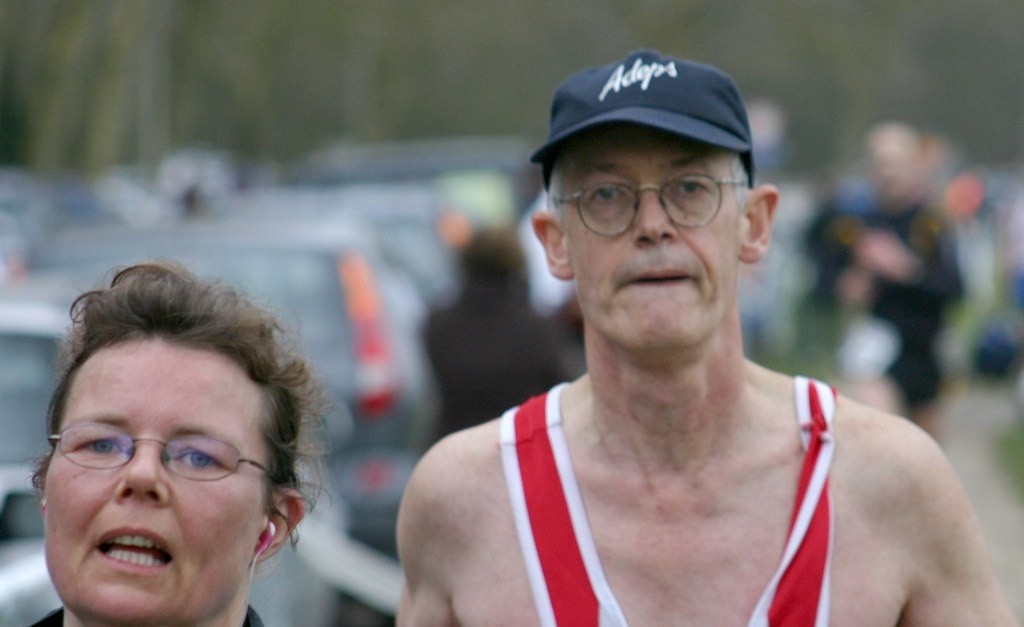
[396,51,1015,625]
[24,264,337,626]
[420,105,974,457]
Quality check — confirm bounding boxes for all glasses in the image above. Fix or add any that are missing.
[550,173,746,237]
[47,418,268,483]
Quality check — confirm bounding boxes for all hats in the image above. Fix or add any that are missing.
[532,51,756,194]
